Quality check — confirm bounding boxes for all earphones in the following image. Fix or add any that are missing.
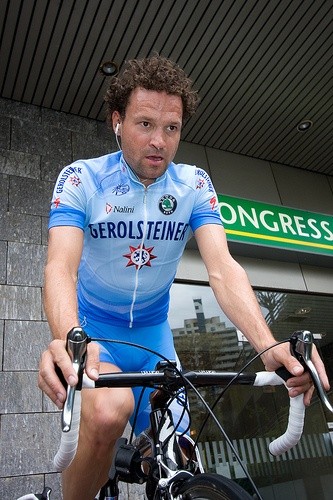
[115,124,120,134]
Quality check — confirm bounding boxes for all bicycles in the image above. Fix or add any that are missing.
[18,327,332,499]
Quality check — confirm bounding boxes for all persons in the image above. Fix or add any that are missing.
[37,56,330,500]
